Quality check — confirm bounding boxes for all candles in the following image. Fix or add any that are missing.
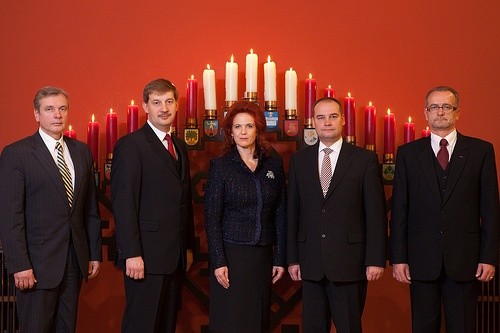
[62,54,237,171]
[245,49,430,164]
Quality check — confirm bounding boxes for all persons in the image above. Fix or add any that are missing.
[109,78,194,333]
[389,84,500,333]
[202,100,286,333]
[0,86,103,333]
[286,97,387,332]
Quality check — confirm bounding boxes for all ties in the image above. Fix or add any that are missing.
[320,148,333,198]
[437,139,449,172]
[55,141,73,208]
[164,134,176,159]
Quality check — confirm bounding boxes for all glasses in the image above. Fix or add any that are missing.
[426,103,458,112]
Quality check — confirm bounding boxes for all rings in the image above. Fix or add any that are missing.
[489,276,493,279]
